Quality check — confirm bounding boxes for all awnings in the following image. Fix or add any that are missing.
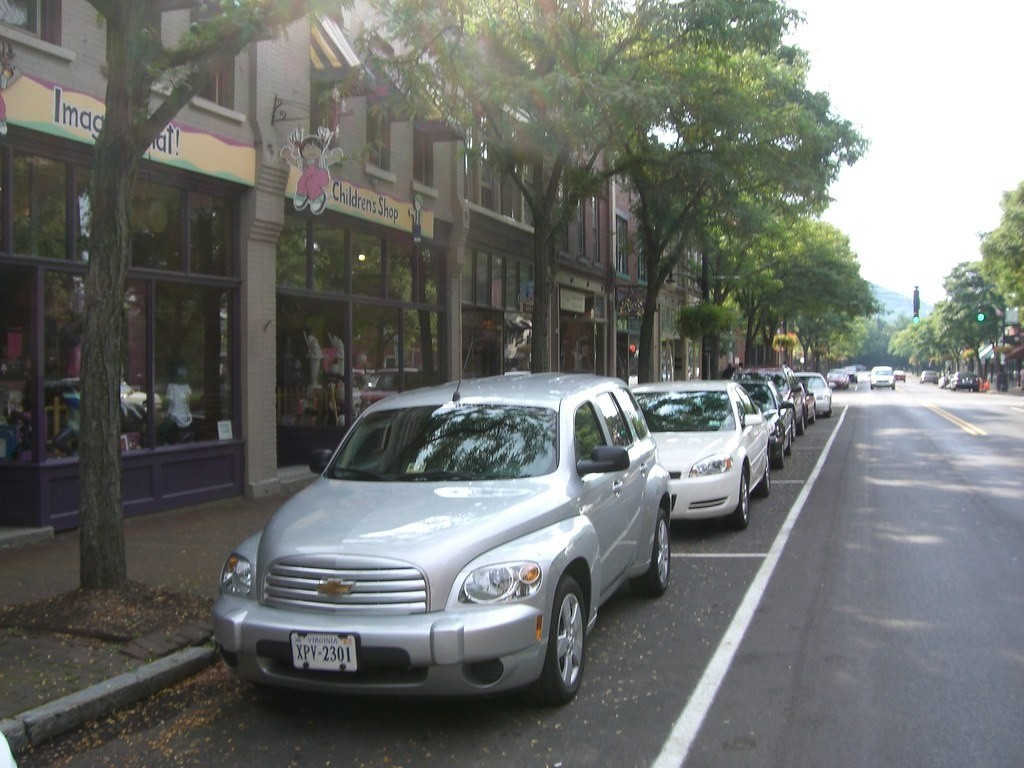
[310,10,376,97]
[367,46,465,142]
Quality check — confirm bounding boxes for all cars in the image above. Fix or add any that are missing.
[331,361,420,425]
[950,371,980,392]
[894,370,906,382]
[869,365,896,390]
[827,369,850,390]
[623,378,774,530]
[207,371,678,705]
[731,377,796,468]
[731,364,818,431]
[49,373,165,429]
[794,371,833,417]
[845,366,859,383]
[919,370,938,385]
[937,375,953,388]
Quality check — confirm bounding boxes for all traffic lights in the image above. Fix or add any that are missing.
[976,303,985,321]
[912,289,921,324]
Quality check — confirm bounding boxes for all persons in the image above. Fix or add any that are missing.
[722,364,736,378]
[166,357,193,428]
